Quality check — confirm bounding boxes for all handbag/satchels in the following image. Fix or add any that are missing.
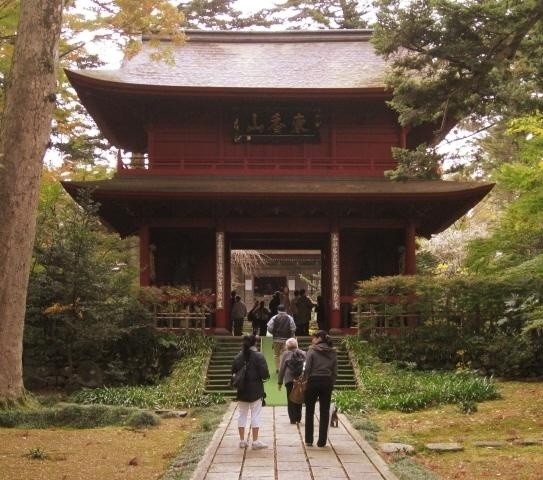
[288,375,310,405]
[230,364,247,390]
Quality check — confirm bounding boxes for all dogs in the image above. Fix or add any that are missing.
[330,407,339,428]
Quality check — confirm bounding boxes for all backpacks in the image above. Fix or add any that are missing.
[284,349,306,377]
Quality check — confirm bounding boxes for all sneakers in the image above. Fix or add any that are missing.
[251,439,268,450]
[239,440,250,448]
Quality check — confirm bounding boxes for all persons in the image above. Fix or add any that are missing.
[229,332,270,451]
[275,337,307,425]
[228,286,325,337]
[267,304,297,374]
[300,329,338,448]
